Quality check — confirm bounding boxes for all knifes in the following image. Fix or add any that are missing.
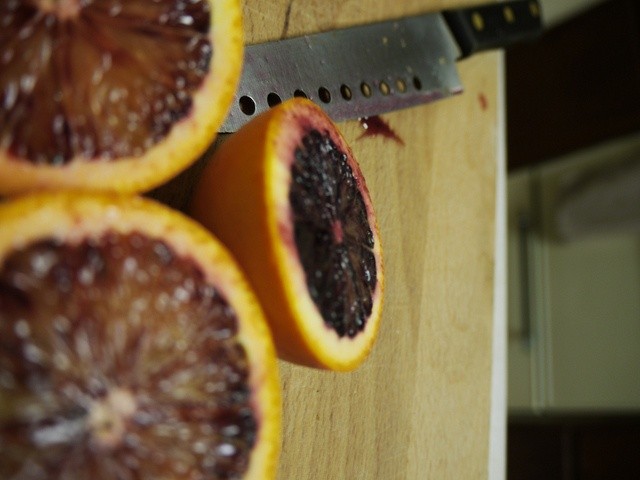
[206,2,546,134]
